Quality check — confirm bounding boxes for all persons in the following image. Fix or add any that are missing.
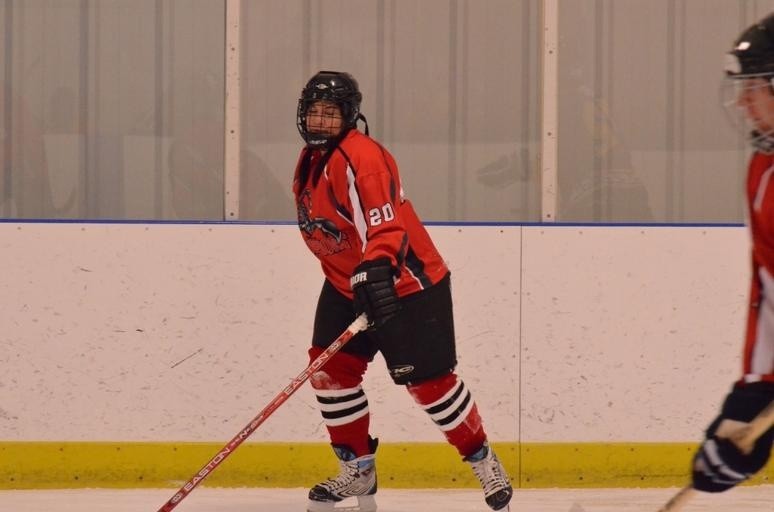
[691,14,774,492]
[292,70,513,510]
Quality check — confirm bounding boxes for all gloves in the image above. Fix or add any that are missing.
[691,381,774,493]
[349,256,403,329]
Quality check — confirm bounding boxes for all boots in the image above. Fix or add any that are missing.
[461,434,514,510]
[308,434,378,504]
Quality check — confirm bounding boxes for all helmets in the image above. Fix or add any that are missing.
[719,14,773,156]
[297,70,362,150]
